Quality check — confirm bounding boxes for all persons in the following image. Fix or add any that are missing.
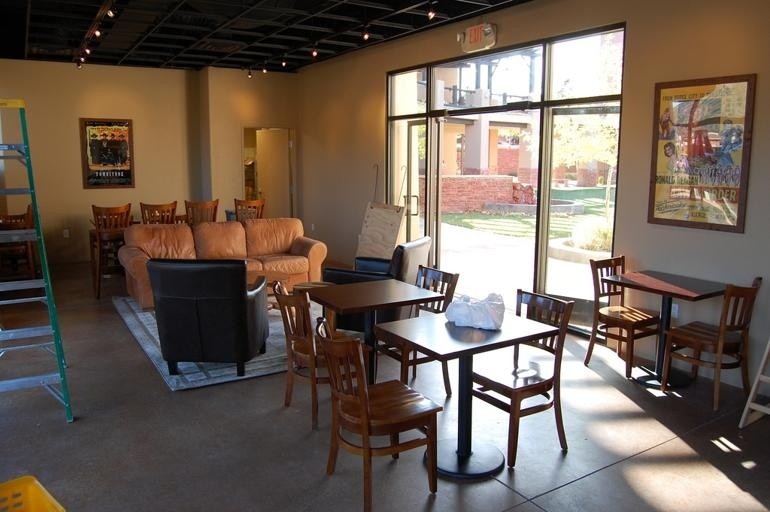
[99,134,110,166]
[89,132,99,164]
[660,109,676,138]
[108,132,119,167]
[663,142,689,177]
[117,134,128,167]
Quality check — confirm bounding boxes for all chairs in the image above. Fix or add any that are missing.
[376,264,460,395]
[659,276,763,410]
[0,198,266,297]
[271,280,351,420]
[316,316,443,511]
[322,235,433,335]
[145,256,269,376]
[472,288,575,469]
[585,255,657,382]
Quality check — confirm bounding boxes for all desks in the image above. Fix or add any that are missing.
[302,278,446,383]
[374,305,546,476]
[601,269,731,388]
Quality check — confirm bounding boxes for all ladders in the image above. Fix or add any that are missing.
[0,99,74,423]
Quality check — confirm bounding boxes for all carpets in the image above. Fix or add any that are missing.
[110,290,381,393]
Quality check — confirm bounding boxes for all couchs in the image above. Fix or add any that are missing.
[117,216,329,291]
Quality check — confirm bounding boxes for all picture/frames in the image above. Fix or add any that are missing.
[79,118,136,190]
[647,76,756,235]
